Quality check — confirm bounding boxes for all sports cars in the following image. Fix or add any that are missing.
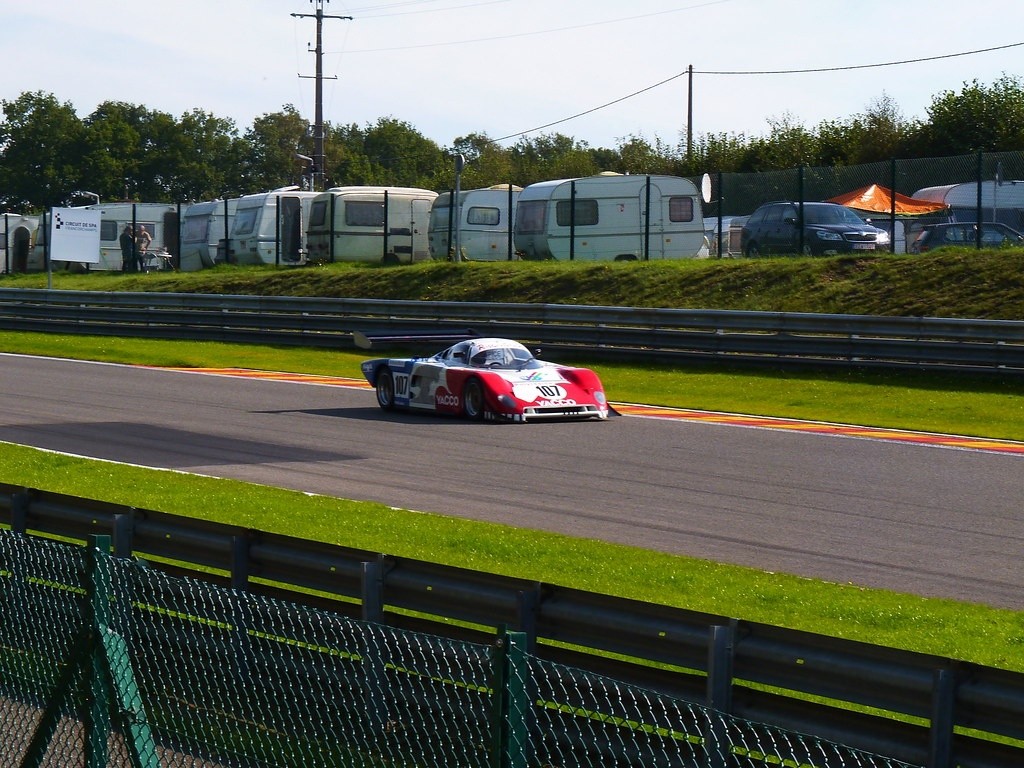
[359,338,609,422]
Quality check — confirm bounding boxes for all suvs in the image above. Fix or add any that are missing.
[740,200,890,256]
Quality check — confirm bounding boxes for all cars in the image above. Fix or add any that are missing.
[907,221,1024,254]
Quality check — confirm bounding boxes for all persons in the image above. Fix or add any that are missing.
[135,225,152,273]
[119,226,132,273]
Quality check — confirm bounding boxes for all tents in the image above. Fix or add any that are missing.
[821,184,949,255]
